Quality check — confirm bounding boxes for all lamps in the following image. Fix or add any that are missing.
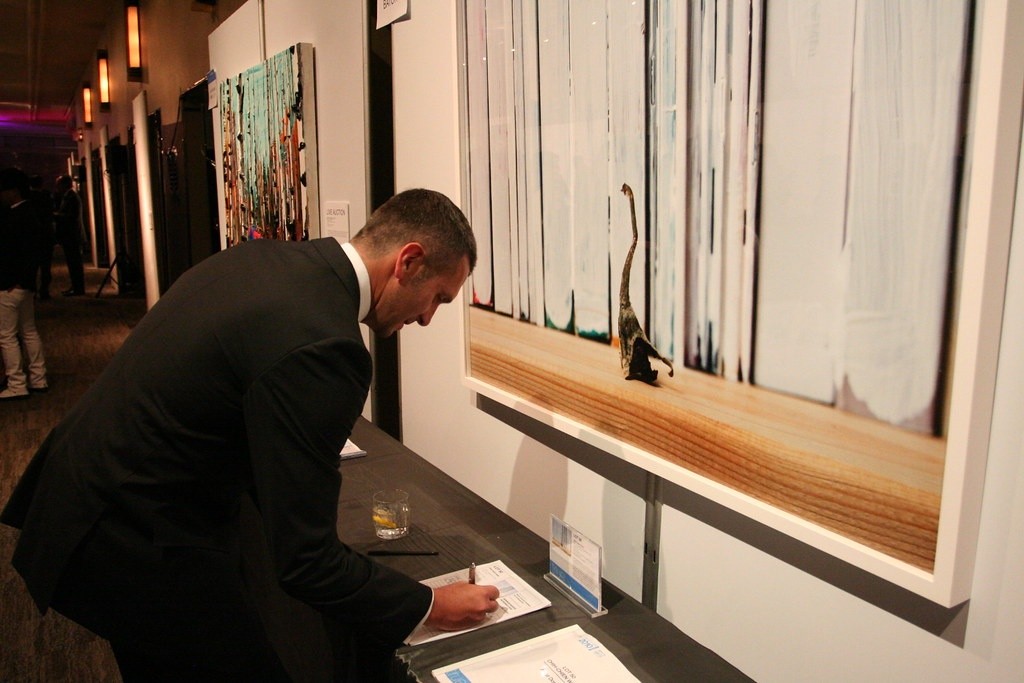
[82,82,92,126]
[96,49,111,113]
[125,5,142,80]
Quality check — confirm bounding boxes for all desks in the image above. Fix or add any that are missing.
[339,415,756,683]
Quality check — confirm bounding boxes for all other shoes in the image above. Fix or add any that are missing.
[62,288,85,297]
[26,377,49,393]
[0,382,30,400]
[39,290,51,300]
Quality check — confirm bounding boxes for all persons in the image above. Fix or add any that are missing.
[0,188,500,683]
[32,175,85,296]
[0,166,49,401]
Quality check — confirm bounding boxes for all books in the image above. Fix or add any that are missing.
[432,624,642,683]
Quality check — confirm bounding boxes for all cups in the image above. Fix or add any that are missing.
[372,487,410,541]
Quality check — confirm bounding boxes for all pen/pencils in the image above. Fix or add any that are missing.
[469,563,476,584]
[368,550,439,557]
